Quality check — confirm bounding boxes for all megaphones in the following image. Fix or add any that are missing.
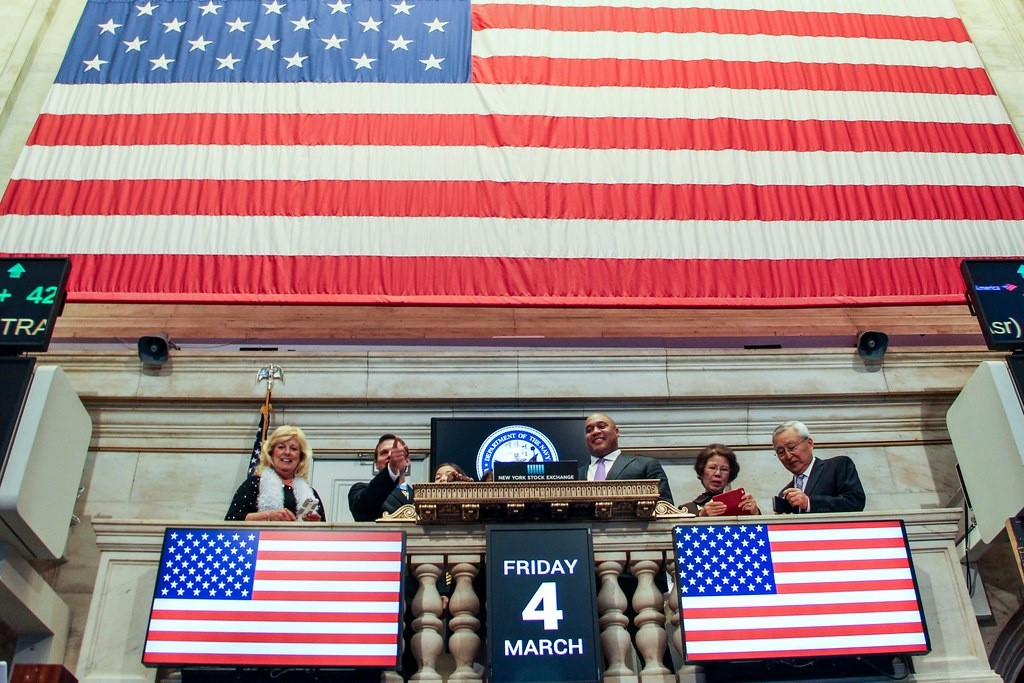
[857,330,889,360]
[137,331,170,365]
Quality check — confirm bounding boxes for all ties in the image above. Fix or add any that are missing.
[795,473,806,514]
[594,458,605,480]
[396,483,409,500]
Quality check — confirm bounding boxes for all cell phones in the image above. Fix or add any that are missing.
[713,487,751,516]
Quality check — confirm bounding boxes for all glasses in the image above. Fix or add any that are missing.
[776,437,808,459]
[705,464,731,476]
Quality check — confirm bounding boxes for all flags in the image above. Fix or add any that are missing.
[674,520,929,661]
[245,380,271,478]
[0,0,1024,298]
[143,527,402,665]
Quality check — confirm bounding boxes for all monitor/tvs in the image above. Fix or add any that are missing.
[494,460,579,483]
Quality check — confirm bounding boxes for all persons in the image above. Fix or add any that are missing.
[578,411,675,675]
[771,420,866,515]
[224,425,326,522]
[678,444,761,516]
[348,433,492,682]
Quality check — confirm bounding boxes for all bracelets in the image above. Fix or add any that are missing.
[267,509,272,521]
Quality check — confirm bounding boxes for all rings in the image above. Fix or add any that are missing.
[749,503,752,506]
